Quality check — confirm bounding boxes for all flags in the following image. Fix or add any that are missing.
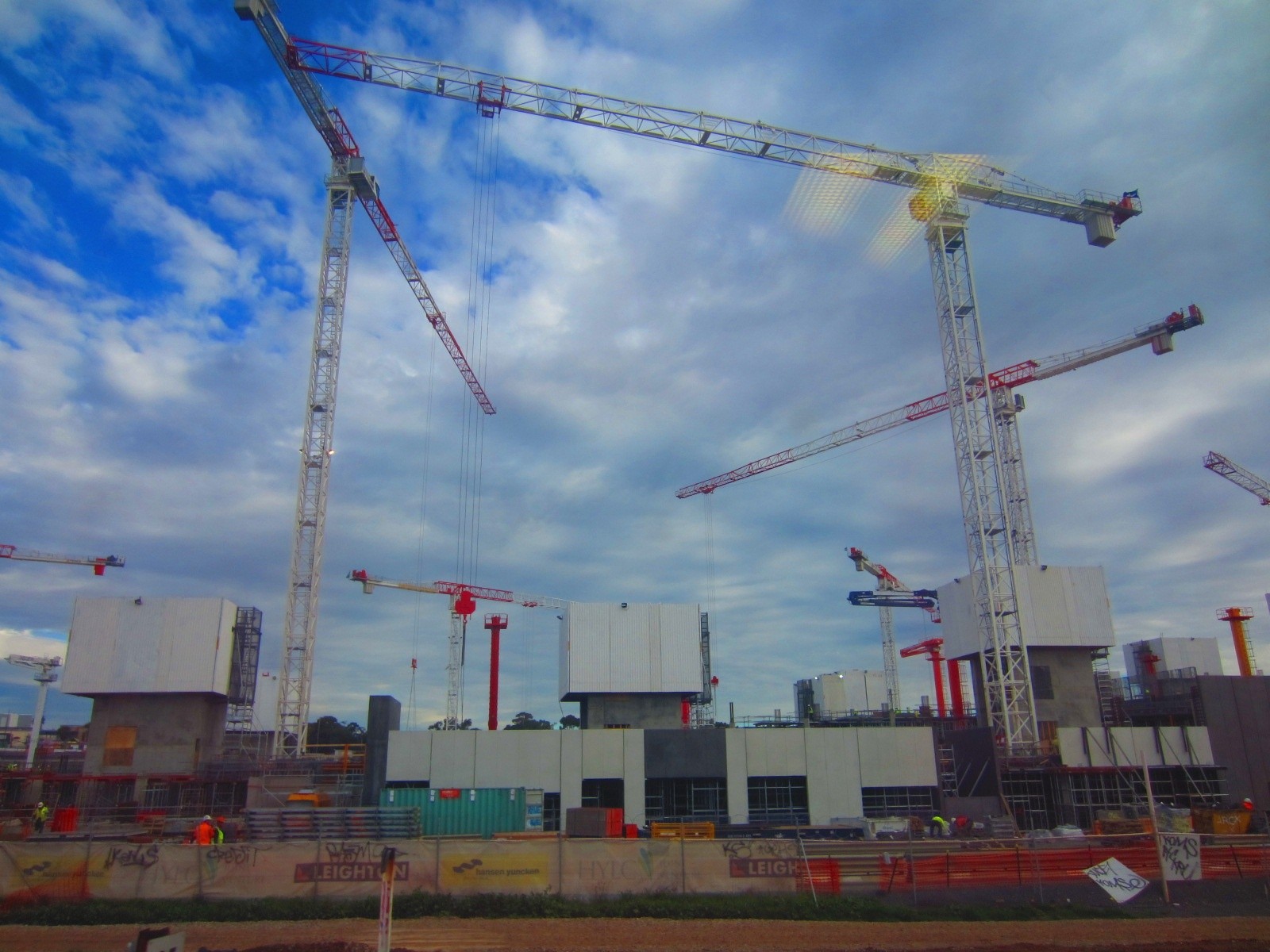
[1123,189,1139,199]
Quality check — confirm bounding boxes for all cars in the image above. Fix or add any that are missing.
[960,816,1085,851]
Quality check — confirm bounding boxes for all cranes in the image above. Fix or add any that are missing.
[228,0,496,757]
[279,30,1143,834]
[676,302,1207,566]
[844,546,941,715]
[0,543,127,576]
[1204,450,1270,507]
[346,569,581,732]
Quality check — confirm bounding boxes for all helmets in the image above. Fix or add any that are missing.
[216,816,225,821]
[1244,798,1251,801]
[204,815,212,820]
[951,818,956,823]
[38,802,43,807]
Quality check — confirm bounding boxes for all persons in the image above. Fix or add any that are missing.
[194,814,225,844]
[32,802,49,833]
[950,816,972,839]
[930,817,947,838]
[1243,797,1254,810]
[807,704,814,721]
[894,706,919,717]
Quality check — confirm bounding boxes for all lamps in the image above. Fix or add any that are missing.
[135,596,141,604]
